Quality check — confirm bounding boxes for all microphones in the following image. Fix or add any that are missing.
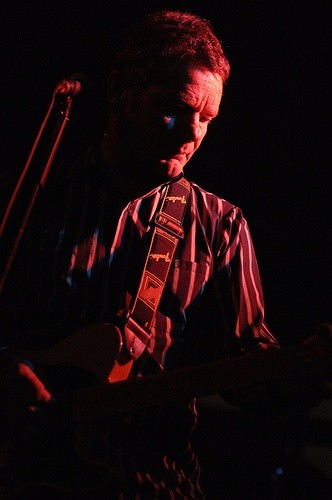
[57,78,80,95]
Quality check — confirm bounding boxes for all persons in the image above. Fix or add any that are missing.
[1,8,330,497]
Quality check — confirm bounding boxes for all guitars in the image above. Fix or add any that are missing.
[3,319,332,500]
